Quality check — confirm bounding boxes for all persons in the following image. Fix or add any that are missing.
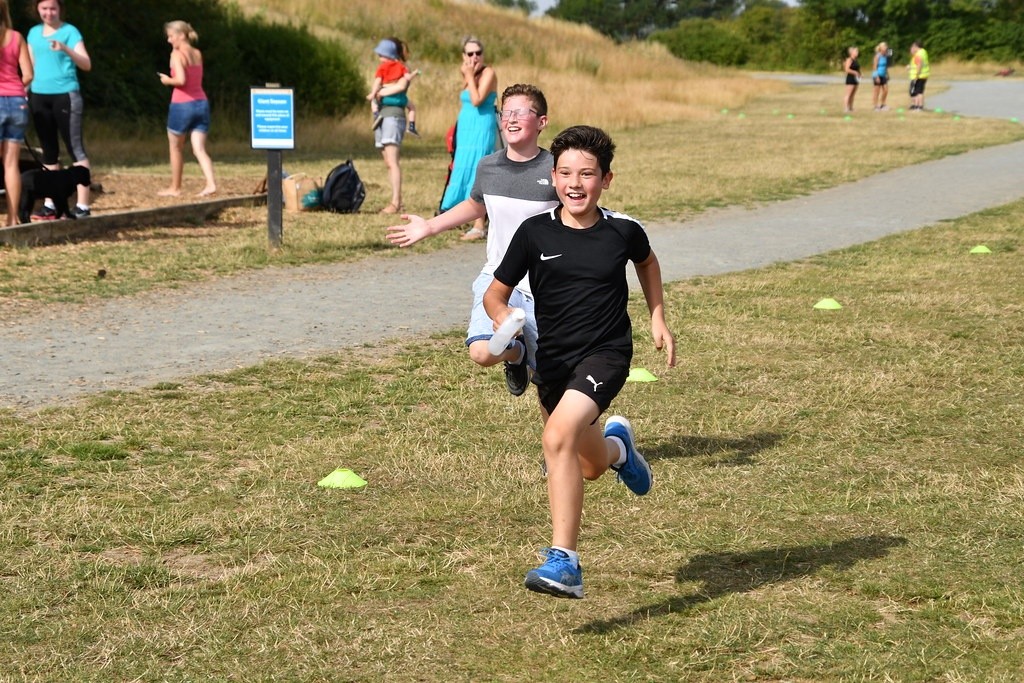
[157,21,217,198]
[441,31,497,242]
[386,84,558,397]
[0,0,91,226]
[844,46,861,112]
[366,36,420,215]
[872,42,890,112]
[905,40,930,111]
[483,124,675,601]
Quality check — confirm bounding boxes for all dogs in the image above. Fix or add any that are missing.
[17,165,91,224]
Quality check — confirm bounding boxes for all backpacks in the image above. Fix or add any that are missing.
[322,159,365,213]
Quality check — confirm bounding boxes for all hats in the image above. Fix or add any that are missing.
[374,39,397,59]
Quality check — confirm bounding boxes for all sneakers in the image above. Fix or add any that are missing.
[60,206,91,220]
[504,334,529,397]
[525,546,583,598]
[29,205,57,219]
[603,416,653,497]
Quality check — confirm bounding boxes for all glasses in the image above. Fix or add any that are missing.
[499,107,538,121]
[465,50,483,57]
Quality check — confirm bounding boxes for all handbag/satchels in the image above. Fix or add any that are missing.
[281,173,322,210]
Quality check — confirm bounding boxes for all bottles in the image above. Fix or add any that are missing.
[487,308,527,356]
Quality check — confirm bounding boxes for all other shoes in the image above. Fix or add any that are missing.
[407,127,420,137]
[371,115,383,131]
[461,227,486,241]
[378,203,404,215]
